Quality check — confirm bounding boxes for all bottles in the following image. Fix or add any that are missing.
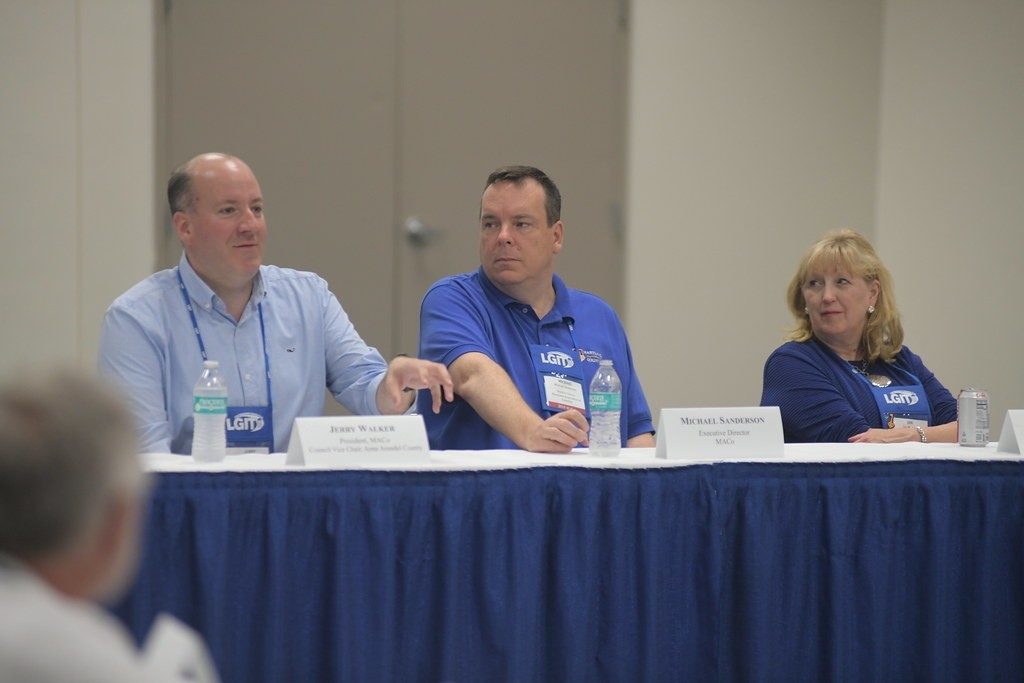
[191,360,227,462]
[588,360,622,457]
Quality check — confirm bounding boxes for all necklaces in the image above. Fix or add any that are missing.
[842,359,870,376]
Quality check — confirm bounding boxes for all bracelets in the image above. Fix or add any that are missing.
[916,425,927,443]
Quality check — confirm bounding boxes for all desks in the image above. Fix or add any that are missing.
[101,440,1024,683]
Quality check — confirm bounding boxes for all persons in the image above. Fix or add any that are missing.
[0,367,222,683]
[104,151,455,457]
[417,165,661,451]
[759,228,958,446]
